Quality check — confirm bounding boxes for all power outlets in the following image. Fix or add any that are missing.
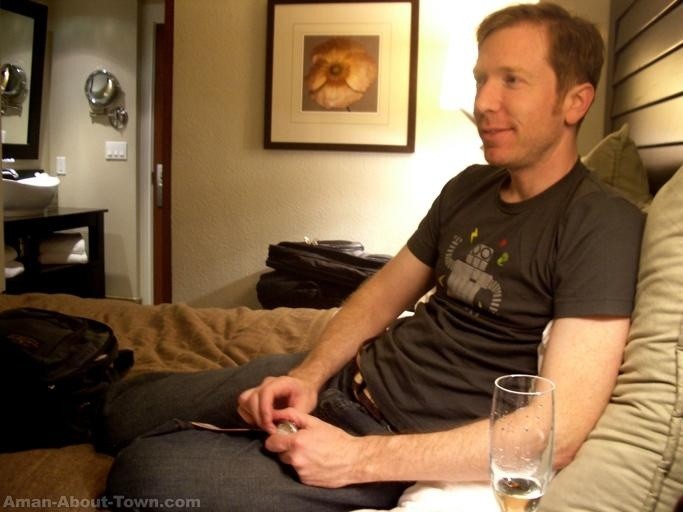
[56,157,66,176]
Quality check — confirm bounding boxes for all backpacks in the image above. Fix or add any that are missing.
[255,232,395,314]
[0,304,136,458]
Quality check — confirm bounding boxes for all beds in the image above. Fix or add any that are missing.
[1,283,682,512]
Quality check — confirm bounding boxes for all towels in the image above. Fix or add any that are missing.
[4,247,25,278]
[40,233,88,266]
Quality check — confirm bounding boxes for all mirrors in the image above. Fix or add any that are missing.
[1,0,49,161]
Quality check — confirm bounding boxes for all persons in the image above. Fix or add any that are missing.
[95,0,644,510]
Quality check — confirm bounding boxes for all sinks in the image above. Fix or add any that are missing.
[2,177,59,218]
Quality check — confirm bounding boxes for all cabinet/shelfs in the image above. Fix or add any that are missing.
[5,213,104,300]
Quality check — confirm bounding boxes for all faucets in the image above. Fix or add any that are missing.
[1,168,19,179]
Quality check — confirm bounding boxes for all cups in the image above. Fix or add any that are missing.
[485,375,558,511]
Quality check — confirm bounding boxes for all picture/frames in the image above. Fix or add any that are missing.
[264,0,420,154]
